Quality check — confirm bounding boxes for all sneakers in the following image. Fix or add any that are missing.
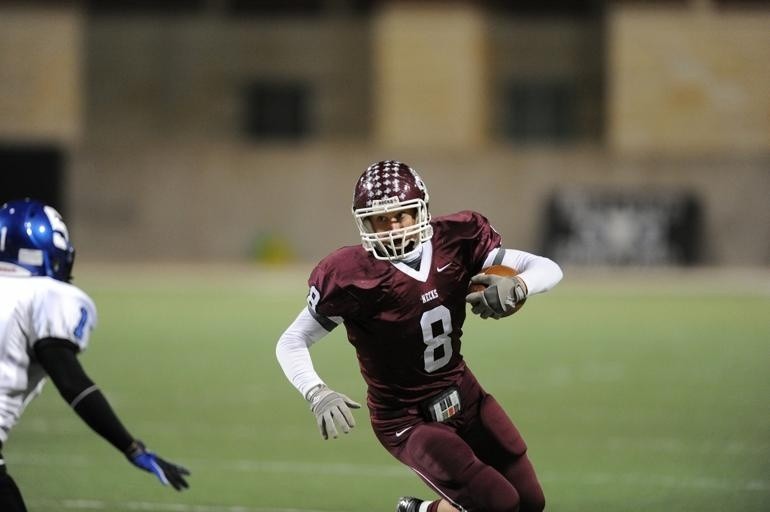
[396,495,423,512]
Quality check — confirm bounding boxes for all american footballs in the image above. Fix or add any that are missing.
[469,264,526,318]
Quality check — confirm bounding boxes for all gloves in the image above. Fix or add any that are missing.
[465,275,527,320]
[305,385,361,440]
[127,440,192,492]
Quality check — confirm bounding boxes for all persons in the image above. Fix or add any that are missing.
[1,196,191,511]
[275,160,564,512]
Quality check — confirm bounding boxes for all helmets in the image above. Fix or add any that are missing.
[0,199,77,283]
[351,160,434,262]
